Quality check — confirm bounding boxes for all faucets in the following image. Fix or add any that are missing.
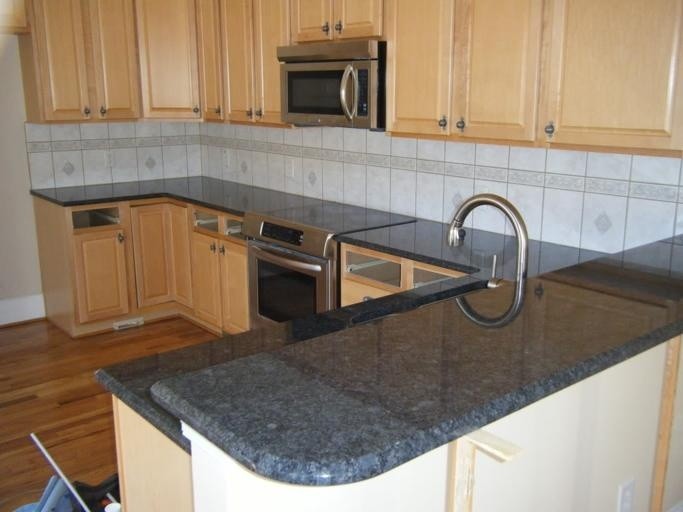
[454,289,527,328]
[445,193,529,289]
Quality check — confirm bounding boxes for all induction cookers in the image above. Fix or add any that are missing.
[245,200,417,234]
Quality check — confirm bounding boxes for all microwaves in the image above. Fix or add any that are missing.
[275,39,386,131]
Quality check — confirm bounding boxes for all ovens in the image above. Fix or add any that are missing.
[240,212,332,334]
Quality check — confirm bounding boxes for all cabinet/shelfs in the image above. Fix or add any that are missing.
[218,3,291,128]
[15,2,144,123]
[133,1,224,121]
[188,204,247,336]
[287,1,391,42]
[541,0,682,156]
[338,243,472,308]
[386,1,545,145]
[31,194,138,339]
